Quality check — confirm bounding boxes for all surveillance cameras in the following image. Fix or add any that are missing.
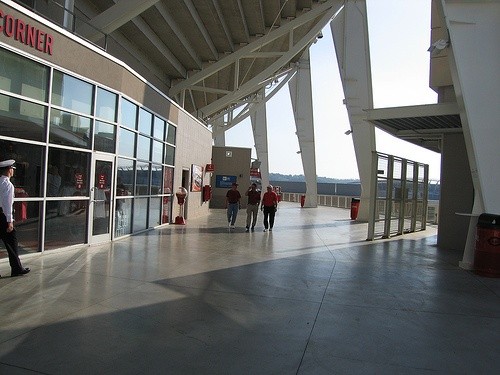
[317,32,323,38]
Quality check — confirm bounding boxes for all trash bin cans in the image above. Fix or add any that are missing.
[473,213,500,277]
[351,198,360,220]
[301,195,305,207]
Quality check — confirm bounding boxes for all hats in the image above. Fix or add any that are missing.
[266,185,273,190]
[232,183,239,186]
[0,159,16,170]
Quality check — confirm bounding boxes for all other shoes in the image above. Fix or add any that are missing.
[245,228,249,232]
[228,221,231,227]
[269,228,273,231]
[231,225,235,229]
[251,228,254,232]
[11,268,30,276]
[263,229,267,232]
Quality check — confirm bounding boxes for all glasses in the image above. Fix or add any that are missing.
[251,186,256,188]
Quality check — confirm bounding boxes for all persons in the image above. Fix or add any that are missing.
[48,166,65,216]
[0,159,31,278]
[245,183,261,232]
[225,183,241,229]
[261,184,278,232]
